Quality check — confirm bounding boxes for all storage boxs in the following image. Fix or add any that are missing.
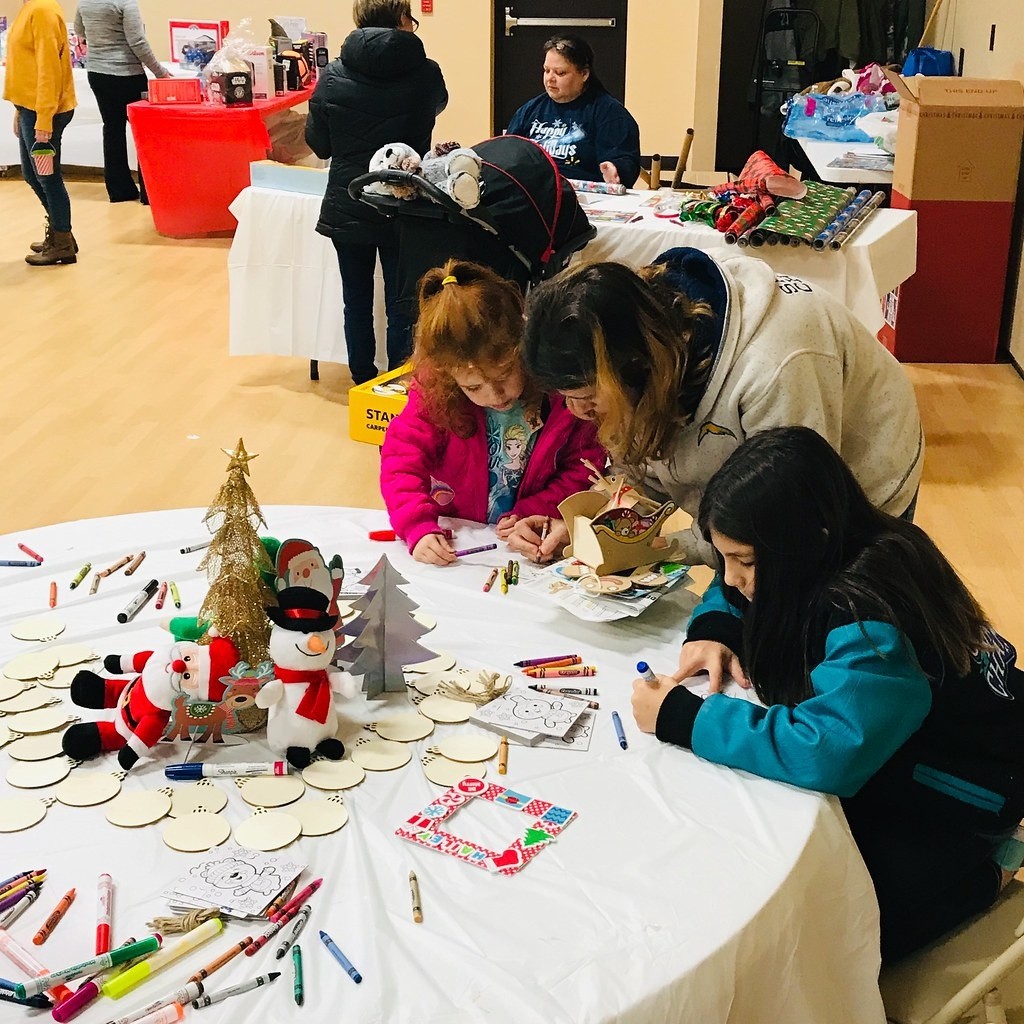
[167,17,230,62]
[879,64,1024,201]
[871,189,1014,366]
[145,78,200,104]
[245,46,275,101]
[346,361,411,445]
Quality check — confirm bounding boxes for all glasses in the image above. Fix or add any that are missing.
[544,40,577,52]
[406,13,419,32]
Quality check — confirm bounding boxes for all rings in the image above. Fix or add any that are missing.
[45,135,48,138]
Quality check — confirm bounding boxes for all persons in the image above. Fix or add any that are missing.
[304,0,449,386]
[379,259,611,567]
[2,0,79,264]
[62,637,238,771]
[73,0,175,206]
[632,426,1024,959]
[507,246,925,569]
[504,33,641,189]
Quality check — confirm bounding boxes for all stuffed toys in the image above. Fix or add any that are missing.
[254,587,356,769]
[363,143,481,210]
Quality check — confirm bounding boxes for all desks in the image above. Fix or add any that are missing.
[0,62,198,179]
[221,148,924,390]
[0,495,893,1024]
[777,92,894,189]
[125,66,319,241]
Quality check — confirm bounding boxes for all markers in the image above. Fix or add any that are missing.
[636,662,659,681]
[106,981,205,1024]
[130,1002,184,1024]
[16,936,160,1000]
[164,760,289,779]
[368,530,456,541]
[117,579,159,624]
[51,950,157,1022]
[101,917,223,997]
[95,873,112,955]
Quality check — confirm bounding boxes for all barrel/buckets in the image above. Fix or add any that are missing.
[30,140,57,176]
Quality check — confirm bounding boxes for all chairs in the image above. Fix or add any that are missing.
[749,4,824,155]
[876,875,1024,1024]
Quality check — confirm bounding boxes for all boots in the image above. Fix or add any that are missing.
[25,225,76,265]
[30,216,78,253]
